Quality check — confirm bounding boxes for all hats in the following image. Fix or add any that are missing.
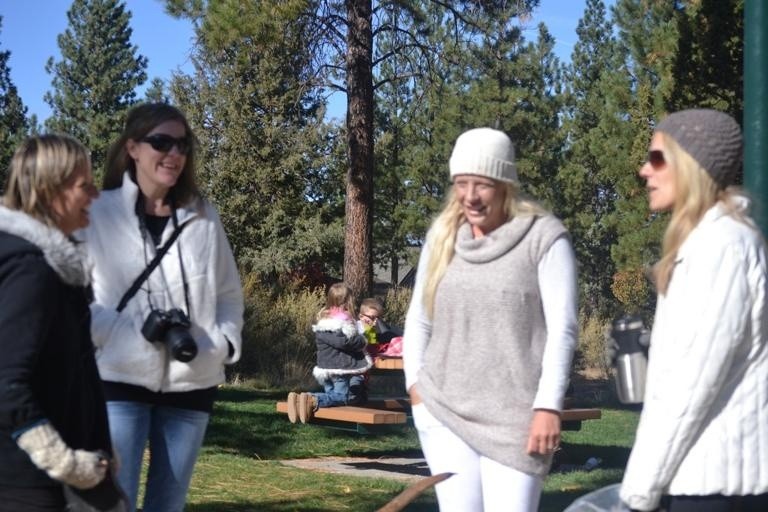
[656,108,743,190]
[448,127,517,183]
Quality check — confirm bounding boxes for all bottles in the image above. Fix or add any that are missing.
[609,317,648,405]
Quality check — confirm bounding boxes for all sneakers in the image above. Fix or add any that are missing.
[288,392,299,423]
[299,392,313,424]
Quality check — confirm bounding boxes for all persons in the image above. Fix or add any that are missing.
[348,297,390,399]
[286,283,375,424]
[618,108,768,512]
[401,127,579,511]
[72,100,245,510]
[0,132,120,511]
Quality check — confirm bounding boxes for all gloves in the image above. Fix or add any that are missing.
[17,423,108,491]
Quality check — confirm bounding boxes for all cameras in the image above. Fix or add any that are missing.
[140,308,197,362]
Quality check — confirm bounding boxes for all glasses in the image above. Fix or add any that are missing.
[363,314,379,321]
[648,149,665,167]
[143,131,191,155]
